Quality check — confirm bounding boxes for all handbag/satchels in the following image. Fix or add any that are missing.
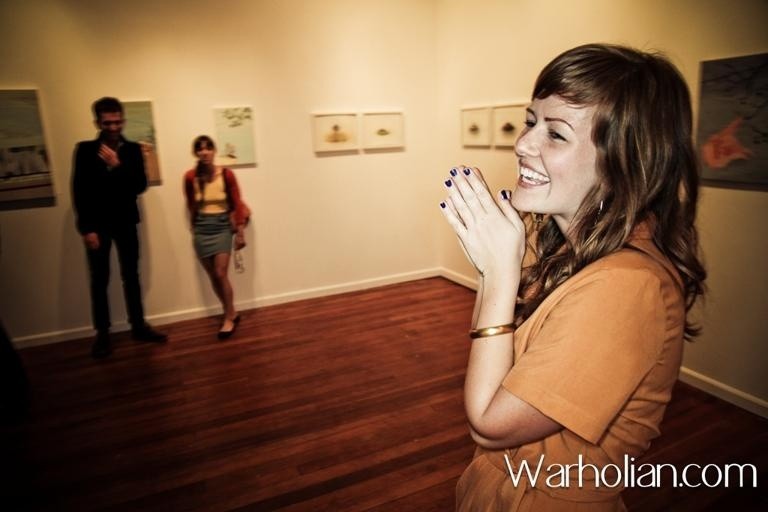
[229,209,250,231]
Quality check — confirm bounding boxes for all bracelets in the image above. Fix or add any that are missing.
[469,324,515,340]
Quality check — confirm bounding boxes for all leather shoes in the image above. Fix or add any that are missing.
[130,326,167,343]
[217,315,241,339]
[92,332,111,355]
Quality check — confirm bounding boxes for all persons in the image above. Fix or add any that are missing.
[183,134,251,341]
[440,43,711,512]
[70,95,168,360]
[1,323,48,511]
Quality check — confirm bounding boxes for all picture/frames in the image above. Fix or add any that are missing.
[312,110,404,155]
[459,104,529,151]
